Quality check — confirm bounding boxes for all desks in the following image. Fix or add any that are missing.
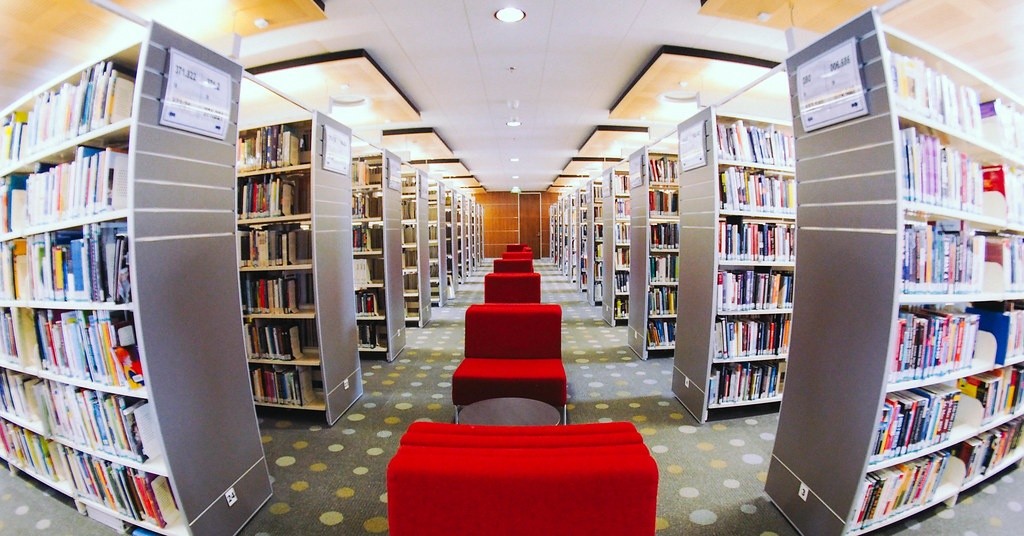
[457,397,561,426]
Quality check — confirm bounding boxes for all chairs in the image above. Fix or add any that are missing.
[387,244,659,536]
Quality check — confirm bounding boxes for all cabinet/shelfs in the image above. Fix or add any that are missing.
[761,4,1024,536]
[0,20,273,535]
[670,105,796,425]
[429,180,484,308]
[629,146,679,360]
[402,168,431,327]
[238,111,363,427]
[602,168,630,327]
[352,148,407,363]
[549,179,603,307]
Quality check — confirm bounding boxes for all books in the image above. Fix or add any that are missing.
[706,123,797,409]
[847,51,1024,536]
[352,159,483,349]
[236,125,313,407]
[0,59,179,536]
[550,150,679,348]
